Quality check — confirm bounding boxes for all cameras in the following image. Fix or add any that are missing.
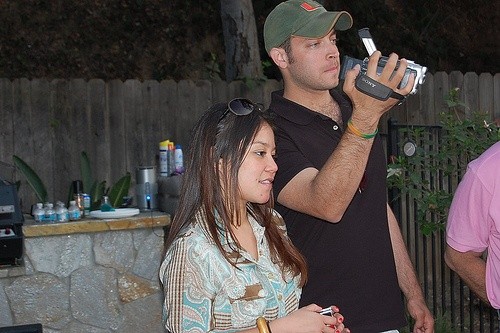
[339,27,428,96]
[318,308,337,332]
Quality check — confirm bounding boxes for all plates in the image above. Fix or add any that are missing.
[90,208,140,218]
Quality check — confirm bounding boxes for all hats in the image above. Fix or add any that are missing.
[263,0,353,56]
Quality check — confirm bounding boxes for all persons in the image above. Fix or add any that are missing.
[259,0,436,333]
[443,140,499,310]
[159,98,350,333]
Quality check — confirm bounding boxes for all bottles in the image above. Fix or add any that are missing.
[32,201,81,223]
[72,179,85,218]
[175,145,184,172]
[167,144,176,176]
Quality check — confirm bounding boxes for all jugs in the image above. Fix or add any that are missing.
[159,139,175,176]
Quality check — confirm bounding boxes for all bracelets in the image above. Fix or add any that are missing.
[347,119,378,138]
[256,317,270,333]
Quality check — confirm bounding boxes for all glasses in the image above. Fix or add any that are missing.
[216,97,265,124]
